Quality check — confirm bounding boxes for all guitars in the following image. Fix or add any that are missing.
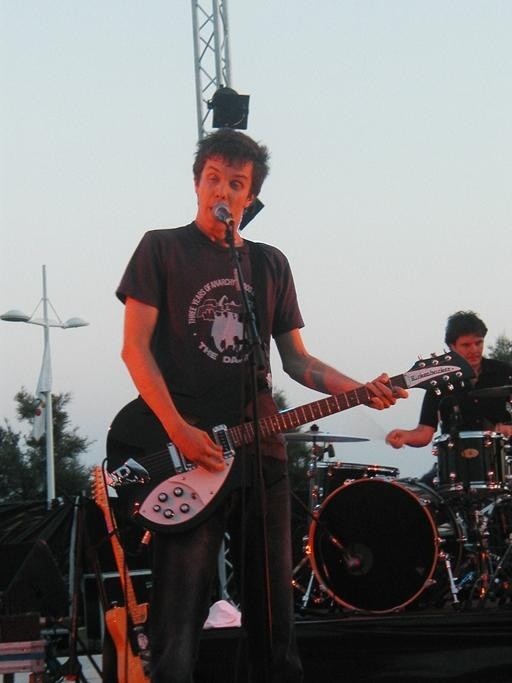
[105,350,475,532]
[89,466,152,682]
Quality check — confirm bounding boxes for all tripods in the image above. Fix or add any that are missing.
[442,502,510,606]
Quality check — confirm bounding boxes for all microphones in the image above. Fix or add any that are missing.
[212,203,235,229]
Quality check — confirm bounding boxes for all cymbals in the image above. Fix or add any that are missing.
[284,429,368,443]
[469,386,512,398]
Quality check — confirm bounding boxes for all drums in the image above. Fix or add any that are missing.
[312,459,395,508]
[443,484,509,539]
[432,430,503,486]
[308,476,458,616]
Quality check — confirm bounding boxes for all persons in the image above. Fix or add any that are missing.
[106,393,244,629]
[385,309,512,449]
[115,127,408,683]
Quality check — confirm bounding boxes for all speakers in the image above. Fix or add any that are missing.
[85,496,153,571]
